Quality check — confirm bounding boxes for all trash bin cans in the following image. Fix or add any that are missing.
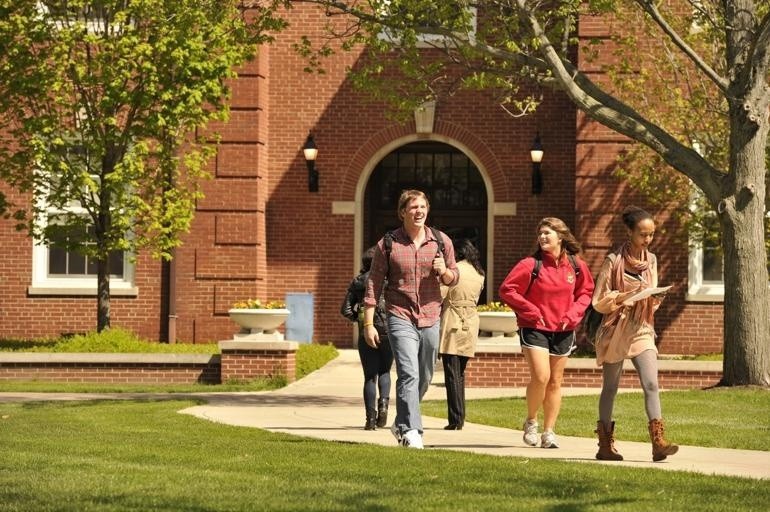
[285,292,313,344]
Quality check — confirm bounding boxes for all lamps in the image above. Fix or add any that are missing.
[529,131,545,194]
[301,130,318,192]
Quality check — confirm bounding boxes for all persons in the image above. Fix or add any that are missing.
[589,204,680,461]
[499,217,596,448]
[340,245,394,431]
[362,190,460,451]
[438,237,486,430]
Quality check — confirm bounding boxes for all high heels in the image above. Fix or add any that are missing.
[444,424,462,431]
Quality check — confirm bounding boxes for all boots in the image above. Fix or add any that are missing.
[595,419,625,461]
[646,418,679,463]
[376,396,392,428]
[363,409,376,431]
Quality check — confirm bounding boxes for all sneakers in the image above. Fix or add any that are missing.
[401,428,428,449]
[540,430,562,448]
[522,418,539,446]
[389,421,405,443]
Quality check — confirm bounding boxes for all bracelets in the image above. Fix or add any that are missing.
[441,268,447,276]
[363,321,373,327]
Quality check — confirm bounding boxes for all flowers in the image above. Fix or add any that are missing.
[228,298,291,309]
[476,301,520,311]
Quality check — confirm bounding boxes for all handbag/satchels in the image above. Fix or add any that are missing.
[582,252,619,346]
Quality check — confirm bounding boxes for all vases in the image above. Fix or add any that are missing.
[227,308,292,341]
[477,311,519,337]
